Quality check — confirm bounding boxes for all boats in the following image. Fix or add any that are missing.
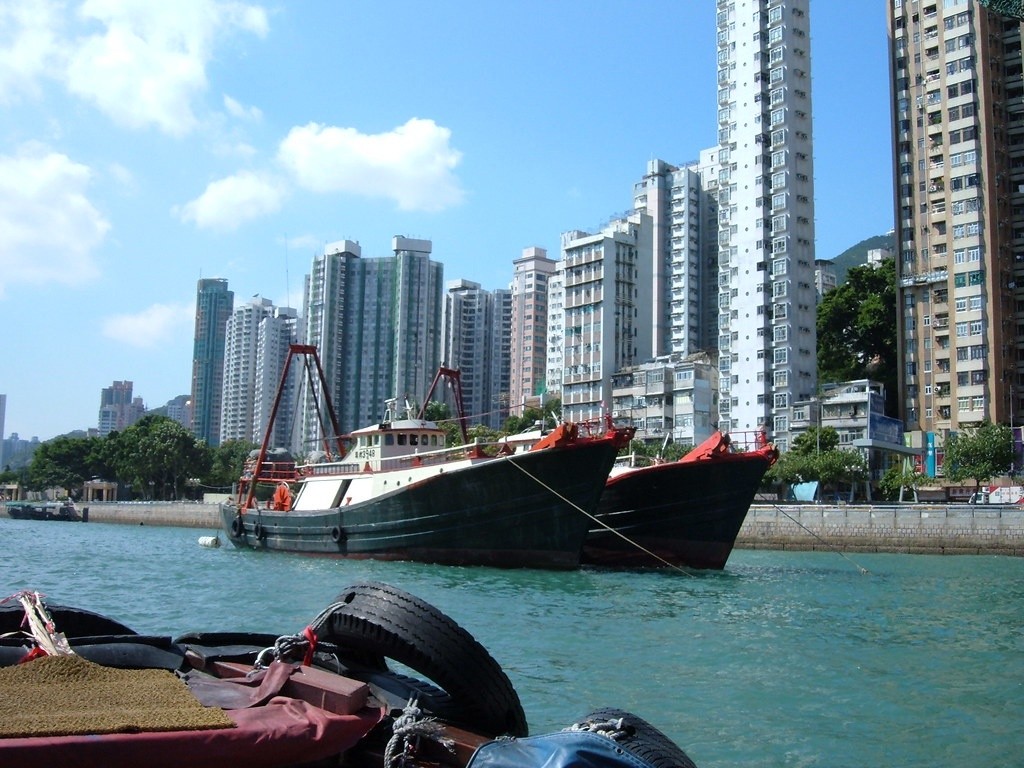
[7,499,79,521]
[579,424,783,572]
[217,332,638,571]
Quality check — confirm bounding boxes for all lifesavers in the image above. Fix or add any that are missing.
[254,523,262,540]
[230,516,243,537]
[558,705,697,768]
[330,525,342,542]
[317,579,532,742]
